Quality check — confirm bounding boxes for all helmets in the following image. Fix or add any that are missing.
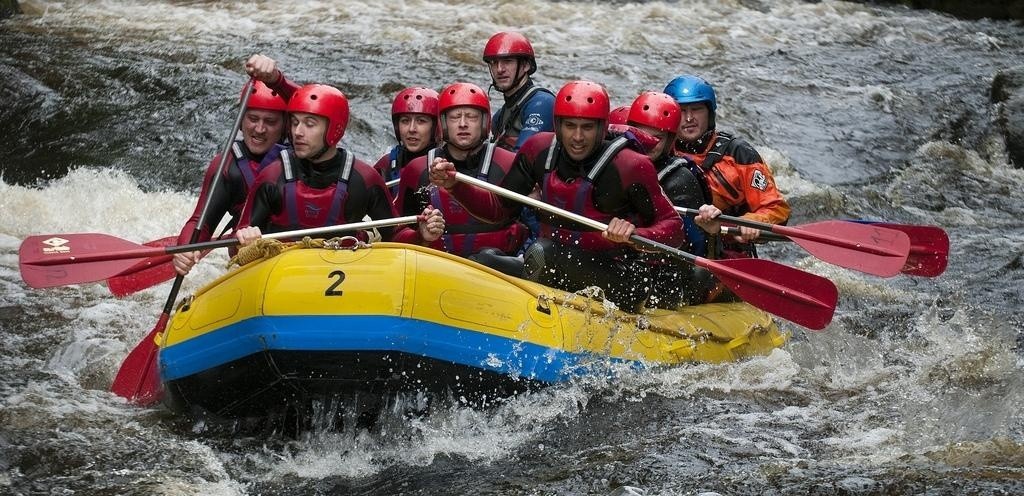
[239,74,288,113]
[608,94,682,136]
[286,82,349,149]
[550,80,613,129]
[482,32,536,62]
[436,82,492,144]
[388,86,443,151]
[658,73,717,113]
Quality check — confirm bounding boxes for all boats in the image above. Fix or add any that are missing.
[152,242,789,438]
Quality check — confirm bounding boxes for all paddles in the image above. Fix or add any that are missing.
[107,176,403,300]
[448,167,839,332]
[108,75,254,399]
[674,204,950,278]
[19,212,432,290]
